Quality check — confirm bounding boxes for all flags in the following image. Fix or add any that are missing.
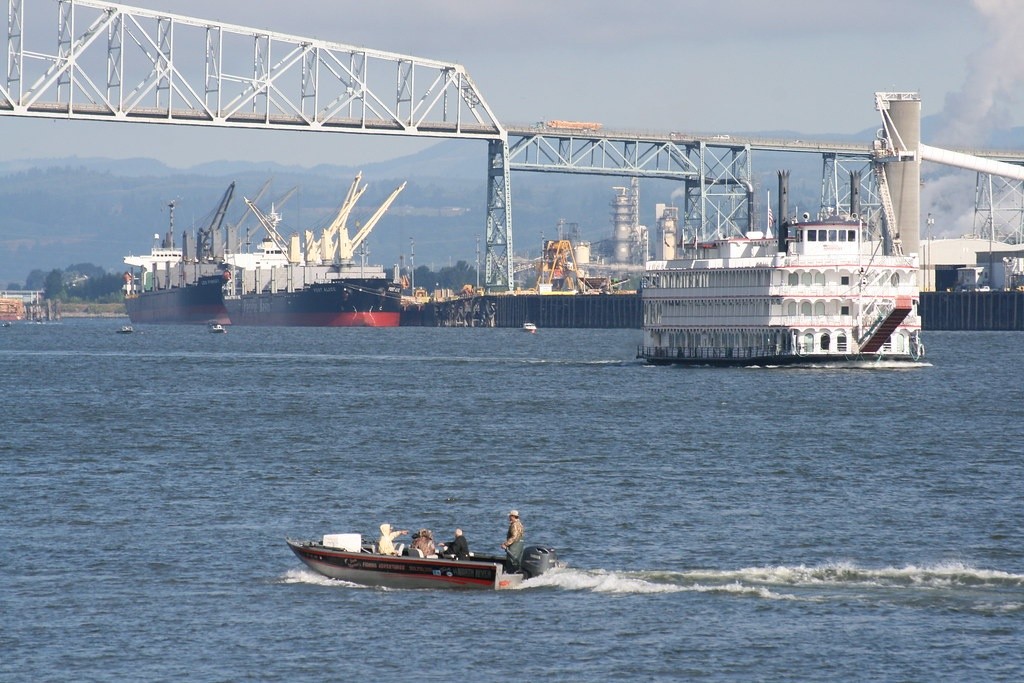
[768,208,777,228]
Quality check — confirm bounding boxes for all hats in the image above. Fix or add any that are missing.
[507,510,519,517]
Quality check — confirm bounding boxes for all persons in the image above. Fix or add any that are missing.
[378,524,409,556]
[438,529,470,561]
[411,529,435,559]
[501,510,525,566]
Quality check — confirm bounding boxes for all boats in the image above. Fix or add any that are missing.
[120,180,299,325]
[523,323,537,335]
[633,168,923,367]
[116,325,134,334]
[2,319,13,327]
[220,170,409,328]
[287,530,559,595]
[207,319,228,334]
